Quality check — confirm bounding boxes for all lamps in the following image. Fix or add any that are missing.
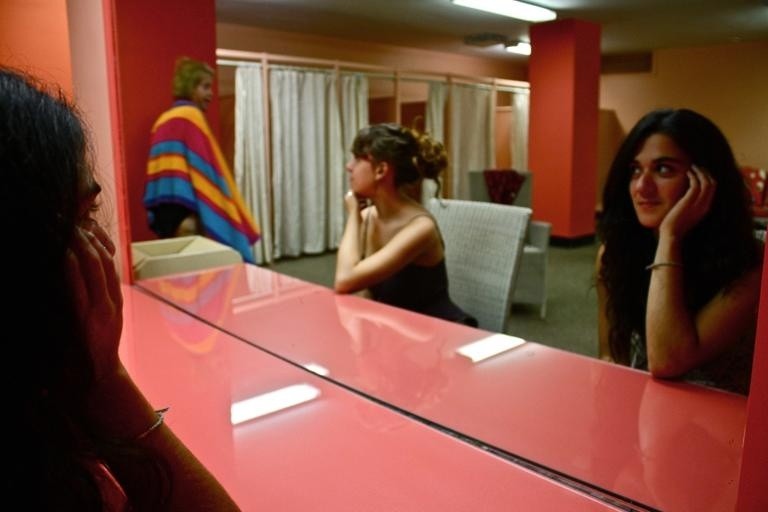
[429,196,533,333]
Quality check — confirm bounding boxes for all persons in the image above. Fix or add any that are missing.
[330,119,480,339]
[586,106,766,400]
[1,60,248,512]
[138,52,264,266]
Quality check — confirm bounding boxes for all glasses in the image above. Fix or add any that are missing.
[504,42,533,56]
[453,0,557,23]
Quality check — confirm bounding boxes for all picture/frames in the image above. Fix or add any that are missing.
[522,221,553,319]
[134,261,748,511]
[118,284,629,511]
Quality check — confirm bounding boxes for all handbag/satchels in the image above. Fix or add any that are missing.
[125,399,171,453]
[645,258,683,275]
